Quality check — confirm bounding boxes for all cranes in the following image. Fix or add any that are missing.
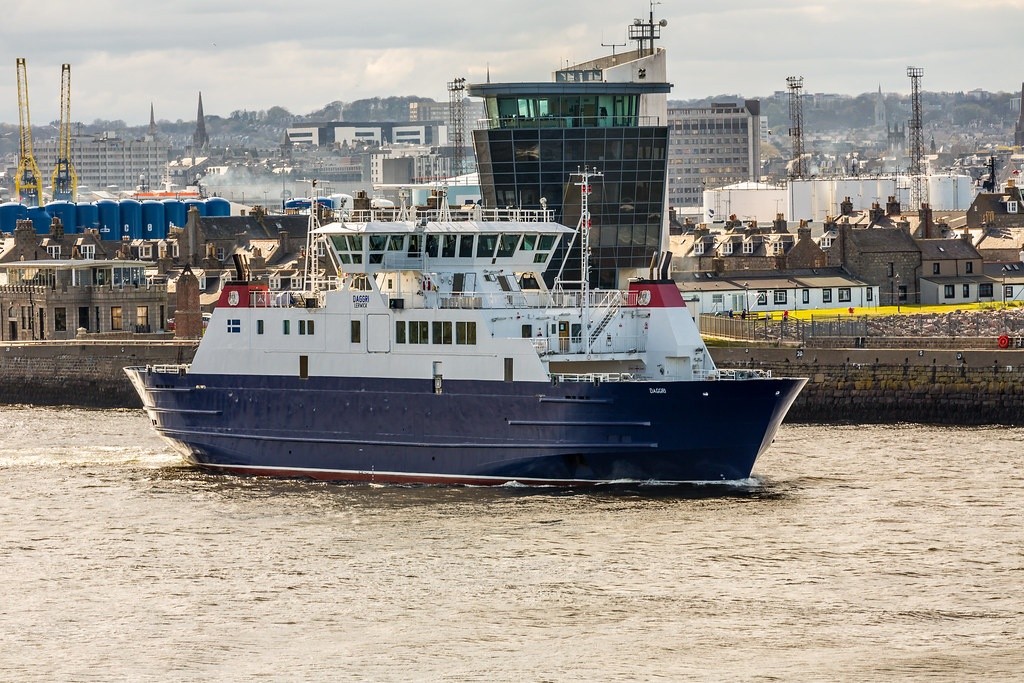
[52,63,78,203]
[15,58,44,207]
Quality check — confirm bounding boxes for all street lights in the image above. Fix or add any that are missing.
[1002,267,1007,304]
[895,273,901,312]
[744,281,749,320]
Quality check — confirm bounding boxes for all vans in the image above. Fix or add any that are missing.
[166,312,213,331]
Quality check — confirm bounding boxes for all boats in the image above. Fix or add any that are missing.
[122,163,810,496]
